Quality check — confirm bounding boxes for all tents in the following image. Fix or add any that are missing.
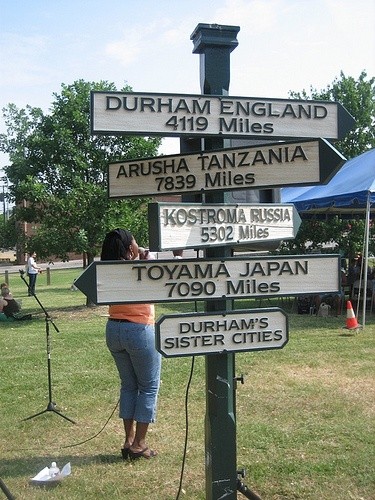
[279,148,375,331]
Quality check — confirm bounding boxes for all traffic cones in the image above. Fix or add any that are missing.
[342,301,363,330]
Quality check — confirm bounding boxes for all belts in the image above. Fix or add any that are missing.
[108,318,130,323]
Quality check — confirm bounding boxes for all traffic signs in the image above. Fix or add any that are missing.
[72,91,357,297]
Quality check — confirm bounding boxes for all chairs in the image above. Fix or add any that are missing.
[352,279,375,314]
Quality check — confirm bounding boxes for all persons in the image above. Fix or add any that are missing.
[100,227,183,458]
[313,255,375,315]
[0,283,13,299]
[0,288,19,322]
[27,251,42,295]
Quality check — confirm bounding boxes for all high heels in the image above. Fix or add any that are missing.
[120,447,129,459]
[128,447,159,461]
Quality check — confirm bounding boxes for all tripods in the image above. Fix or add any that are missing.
[18,274,78,425]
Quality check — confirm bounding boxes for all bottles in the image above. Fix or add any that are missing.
[49,462,60,479]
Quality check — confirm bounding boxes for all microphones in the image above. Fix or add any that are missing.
[137,246,151,255]
